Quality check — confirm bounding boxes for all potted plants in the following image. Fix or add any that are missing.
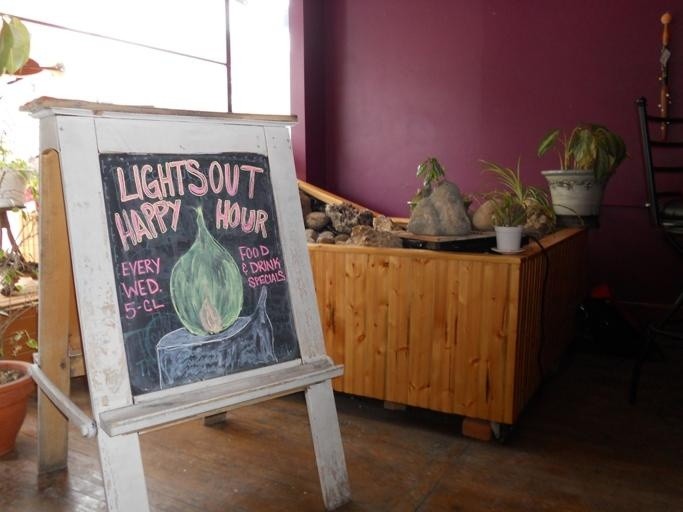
[537,125,627,218]
[489,192,533,252]
[408,155,447,216]
[480,154,589,230]
[0,254,62,462]
[1,151,39,296]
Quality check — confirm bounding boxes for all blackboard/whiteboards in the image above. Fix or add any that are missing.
[99,153,302,396]
